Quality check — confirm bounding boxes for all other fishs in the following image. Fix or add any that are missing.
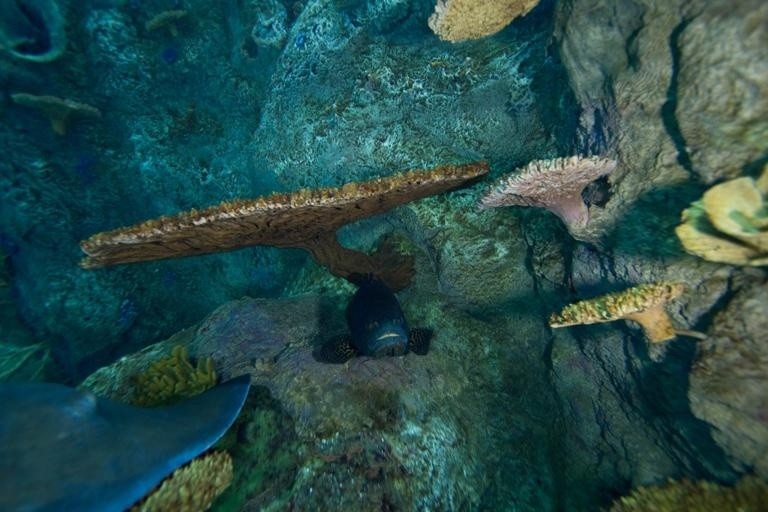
[0,371,252,512]
[319,270,434,365]
